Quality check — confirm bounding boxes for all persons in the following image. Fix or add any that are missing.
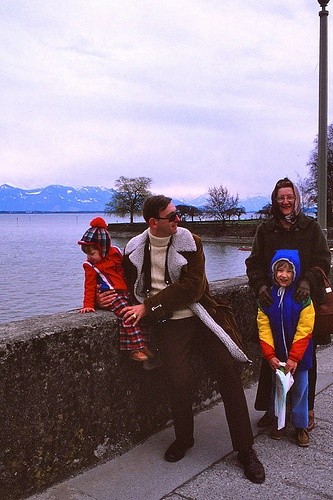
[257,250,315,445]
[245,178,331,431]
[77,216,153,360]
[93,195,265,483]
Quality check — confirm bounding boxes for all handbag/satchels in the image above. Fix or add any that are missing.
[274,362,294,430]
[308,267,333,335]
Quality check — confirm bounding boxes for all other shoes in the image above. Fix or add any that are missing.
[258,411,275,427]
[307,411,315,430]
[271,422,288,440]
[296,429,310,446]
[128,347,155,361]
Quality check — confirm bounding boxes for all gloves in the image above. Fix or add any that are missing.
[259,285,275,308]
[293,278,310,303]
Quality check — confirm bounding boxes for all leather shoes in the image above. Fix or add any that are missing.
[164,439,195,462]
[238,448,265,483]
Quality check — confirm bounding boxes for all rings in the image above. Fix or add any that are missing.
[134,315,138,318]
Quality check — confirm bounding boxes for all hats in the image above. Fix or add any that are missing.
[78,217,111,258]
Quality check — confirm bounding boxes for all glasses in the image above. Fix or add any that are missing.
[275,194,299,203]
[152,209,182,223]
